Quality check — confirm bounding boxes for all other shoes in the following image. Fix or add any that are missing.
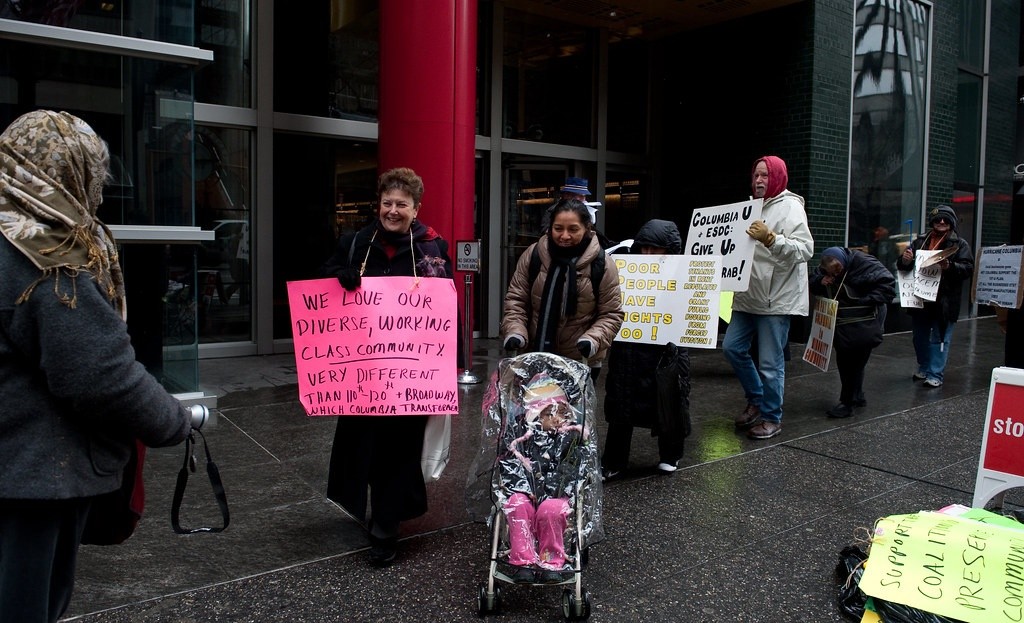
[855,392,867,407]
[826,403,853,419]
[659,461,681,471]
[513,564,537,584]
[600,468,619,480]
[358,523,398,561]
[534,569,565,584]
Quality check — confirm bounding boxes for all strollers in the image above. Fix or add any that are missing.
[476,344,592,621]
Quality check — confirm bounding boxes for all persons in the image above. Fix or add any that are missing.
[498,198,626,407]
[722,156,814,439]
[325,167,454,561]
[599,220,690,483]
[808,247,896,417]
[896,204,974,388]
[0,110,191,623]
[497,373,586,584]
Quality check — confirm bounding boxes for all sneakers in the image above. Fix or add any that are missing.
[750,420,783,438]
[911,372,927,380]
[922,377,943,387]
[734,405,764,427]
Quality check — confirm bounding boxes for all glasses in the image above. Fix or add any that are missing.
[934,217,950,226]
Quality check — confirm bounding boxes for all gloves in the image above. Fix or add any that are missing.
[503,337,521,352]
[146,407,192,448]
[746,223,776,248]
[334,268,361,292]
[577,340,594,359]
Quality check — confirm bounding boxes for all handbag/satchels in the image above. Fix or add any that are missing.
[825,281,884,347]
[78,439,147,546]
[420,413,454,482]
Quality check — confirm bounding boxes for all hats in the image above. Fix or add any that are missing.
[559,177,591,196]
[928,204,958,230]
[509,370,591,469]
